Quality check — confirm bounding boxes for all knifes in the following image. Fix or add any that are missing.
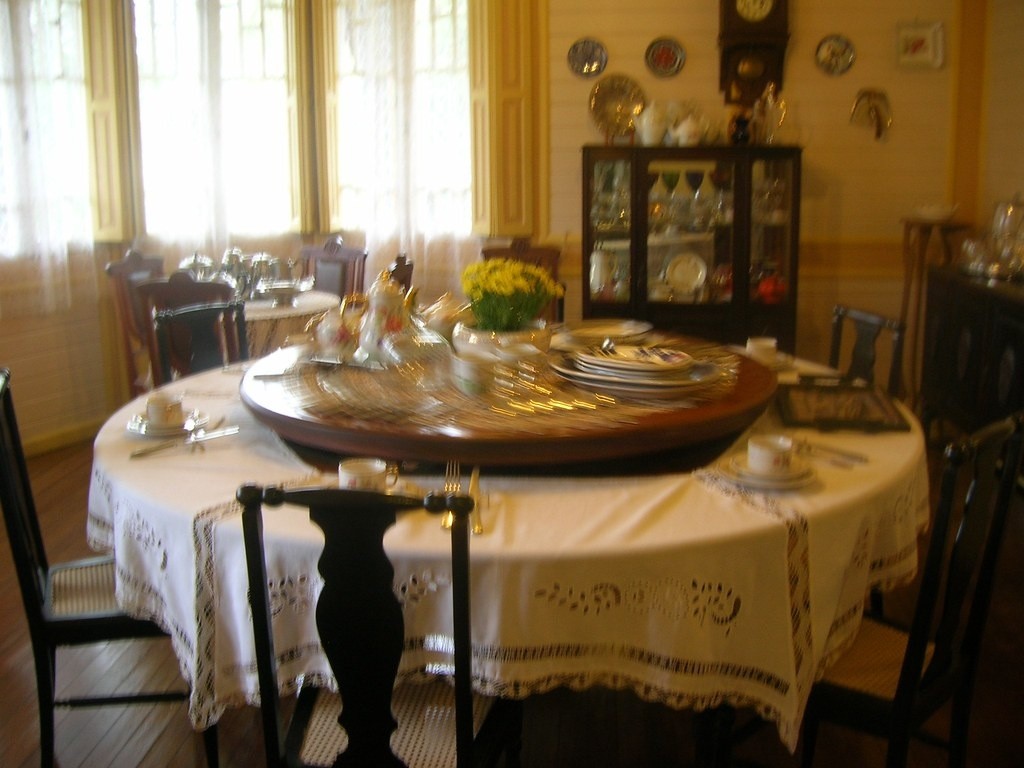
[468,465,482,537]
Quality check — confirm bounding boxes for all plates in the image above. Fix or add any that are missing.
[730,451,810,481]
[666,253,707,297]
[567,37,608,78]
[546,346,719,400]
[717,457,817,491]
[126,409,209,440]
[644,35,687,77]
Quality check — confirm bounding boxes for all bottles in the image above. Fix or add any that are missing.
[649,169,718,228]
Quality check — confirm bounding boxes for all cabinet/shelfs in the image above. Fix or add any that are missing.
[922,268,1024,489]
[580,144,804,358]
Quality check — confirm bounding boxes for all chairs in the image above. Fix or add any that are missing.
[828,304,907,398]
[137,270,238,387]
[156,298,248,387]
[387,253,414,297]
[236,483,503,768]
[0,367,219,768]
[482,236,559,324]
[298,238,367,312]
[694,417,1015,768]
[106,249,163,400]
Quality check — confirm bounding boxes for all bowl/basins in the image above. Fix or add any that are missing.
[564,318,652,347]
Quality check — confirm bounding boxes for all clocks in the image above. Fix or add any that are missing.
[719,0,791,107]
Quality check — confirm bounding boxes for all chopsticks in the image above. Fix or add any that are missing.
[131,427,240,460]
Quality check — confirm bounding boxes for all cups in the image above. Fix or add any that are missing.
[338,459,399,494]
[746,336,777,363]
[747,435,793,478]
[144,392,184,431]
[985,200,1024,281]
[450,349,501,396]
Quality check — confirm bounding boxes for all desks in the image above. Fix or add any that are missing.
[159,277,342,359]
[87,315,932,768]
[895,216,974,411]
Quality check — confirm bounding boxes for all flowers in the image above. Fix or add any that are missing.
[459,256,564,332]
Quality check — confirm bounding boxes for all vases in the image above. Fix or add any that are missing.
[452,319,550,358]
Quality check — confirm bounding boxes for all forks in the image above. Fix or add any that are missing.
[441,460,461,530]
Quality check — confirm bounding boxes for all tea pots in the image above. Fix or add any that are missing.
[667,114,710,147]
[339,269,420,360]
[632,98,677,147]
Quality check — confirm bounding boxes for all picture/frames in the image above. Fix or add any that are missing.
[895,18,943,69]
